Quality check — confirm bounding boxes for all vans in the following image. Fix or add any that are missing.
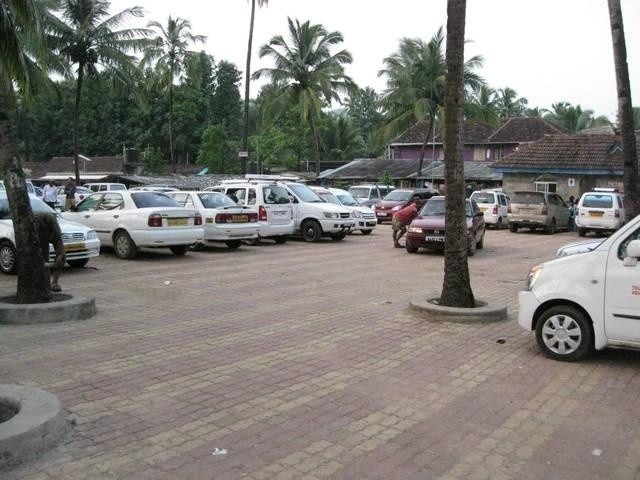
[574,185,625,238]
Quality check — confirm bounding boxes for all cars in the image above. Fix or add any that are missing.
[515,209,640,366]
[504,188,572,235]
[555,238,608,261]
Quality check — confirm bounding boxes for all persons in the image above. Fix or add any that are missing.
[44,178,59,210]
[566,195,579,231]
[64,176,76,209]
[34,210,66,292]
[392,197,422,248]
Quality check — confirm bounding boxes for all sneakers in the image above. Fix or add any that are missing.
[51,283,61,291]
